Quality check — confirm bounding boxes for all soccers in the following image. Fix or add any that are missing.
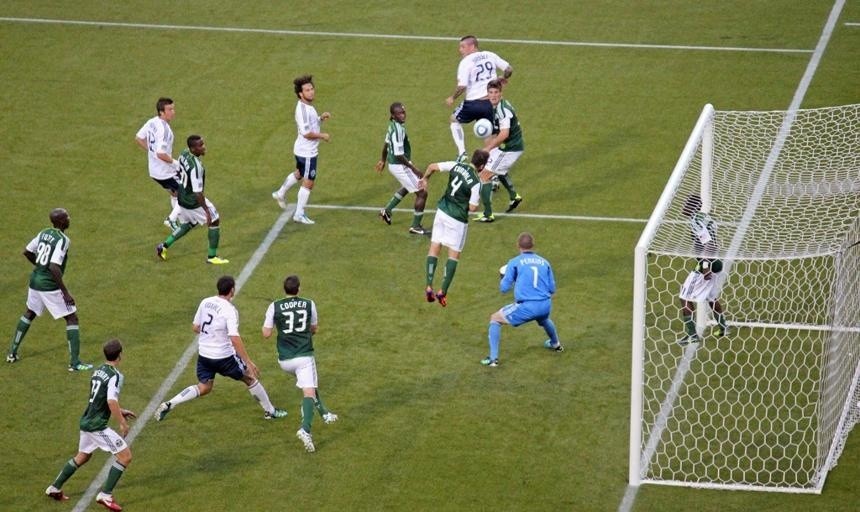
[473,117,493,138]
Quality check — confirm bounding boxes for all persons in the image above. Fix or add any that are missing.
[153,275,288,421]
[134,97,181,232]
[5,207,94,372]
[416,148,490,306]
[471,78,524,222]
[45,337,137,512]
[271,75,331,226]
[678,196,731,345]
[479,231,565,368]
[374,102,431,235]
[260,275,339,453]
[156,135,231,265]
[444,35,514,164]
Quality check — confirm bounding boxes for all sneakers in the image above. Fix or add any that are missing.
[157,219,229,264]
[6,353,20,362]
[456,151,469,163]
[46,484,70,501]
[67,363,93,371]
[425,286,447,306]
[481,357,498,367]
[380,195,522,233]
[272,190,287,209]
[96,491,123,511]
[154,401,171,421]
[266,410,339,451]
[680,331,730,341]
[544,339,563,352]
